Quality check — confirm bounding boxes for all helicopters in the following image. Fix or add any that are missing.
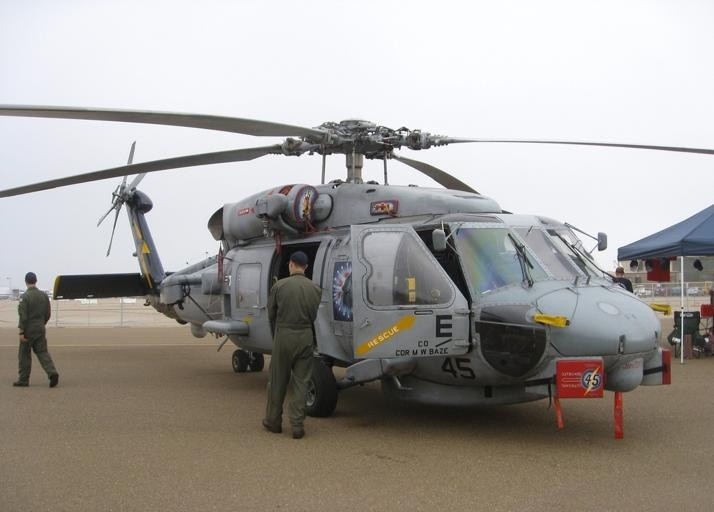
[0,101,714,423]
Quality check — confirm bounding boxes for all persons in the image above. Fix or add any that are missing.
[12,271,59,387]
[261,249,323,439]
[611,266,634,293]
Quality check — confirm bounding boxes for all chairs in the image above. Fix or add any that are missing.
[668,310,706,359]
[697,304,714,333]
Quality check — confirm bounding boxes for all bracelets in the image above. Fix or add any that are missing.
[18,332,25,336]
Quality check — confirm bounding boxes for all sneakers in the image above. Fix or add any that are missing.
[13,382,29,386]
[292,426,305,439]
[262,418,282,434]
[50,373,58,387]
